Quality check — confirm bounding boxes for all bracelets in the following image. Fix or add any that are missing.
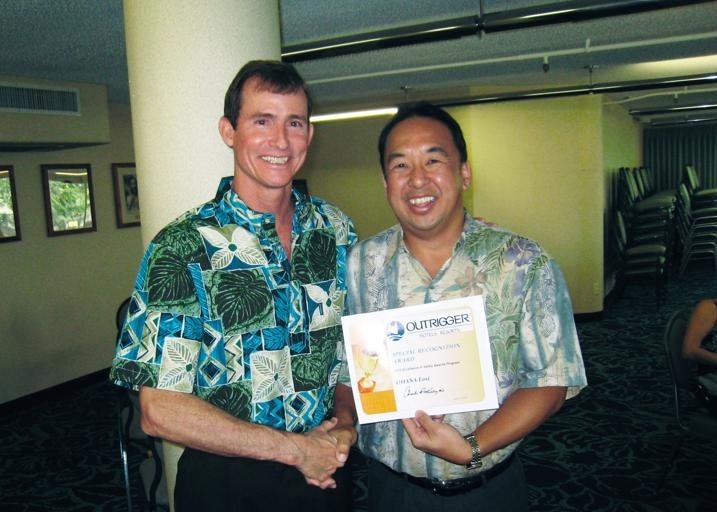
[464,434,484,471]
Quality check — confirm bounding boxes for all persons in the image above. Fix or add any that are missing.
[110,60,499,512]
[304,100,591,511]
[681,294,715,416]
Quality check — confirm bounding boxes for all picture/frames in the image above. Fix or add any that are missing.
[0,162,143,242]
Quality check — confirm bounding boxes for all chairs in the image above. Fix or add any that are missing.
[112,296,160,511]
[605,167,716,452]
